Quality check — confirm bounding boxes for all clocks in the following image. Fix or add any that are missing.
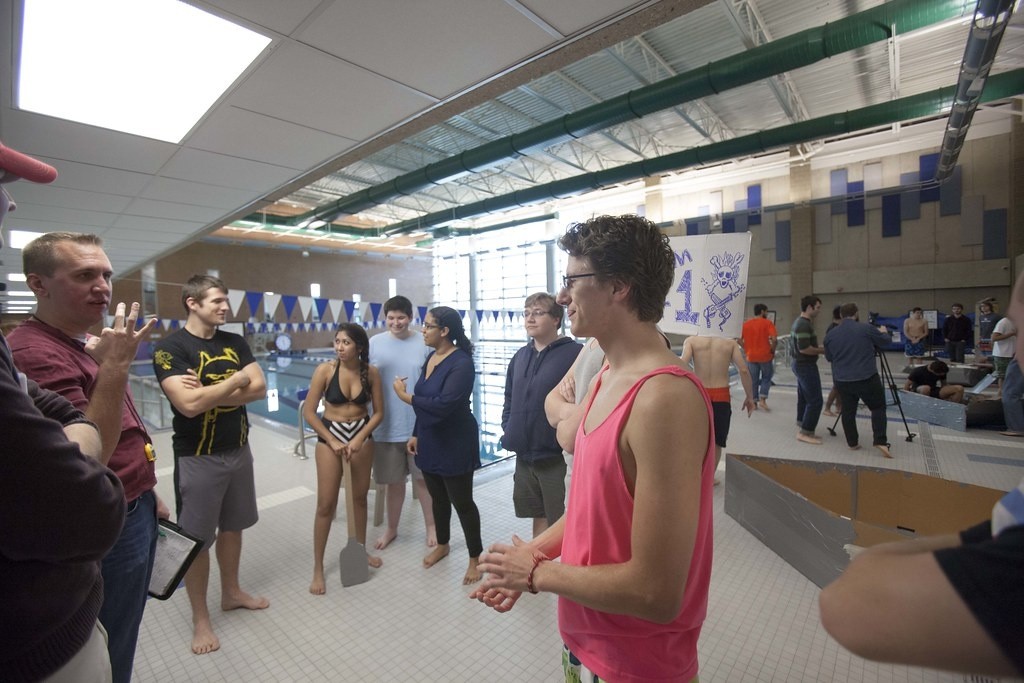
[275,333,292,356]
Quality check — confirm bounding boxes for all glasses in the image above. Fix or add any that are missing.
[423,321,443,330]
[522,309,553,318]
[563,273,596,288]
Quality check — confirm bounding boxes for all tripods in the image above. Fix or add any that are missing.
[827,344,917,442]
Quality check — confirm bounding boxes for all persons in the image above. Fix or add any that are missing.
[978,301,1003,355]
[788,295,825,445]
[367,295,436,550]
[499,293,671,539]
[823,304,895,458]
[153,275,271,656]
[302,322,383,595]
[0,142,170,683]
[903,307,929,365]
[470,214,716,683]
[736,304,777,412]
[942,303,972,364]
[393,306,484,586]
[679,335,755,485]
[818,269,1024,683]
[904,361,964,403]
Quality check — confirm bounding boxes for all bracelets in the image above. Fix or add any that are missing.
[527,553,550,594]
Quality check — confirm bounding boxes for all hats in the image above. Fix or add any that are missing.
[0,142,57,184]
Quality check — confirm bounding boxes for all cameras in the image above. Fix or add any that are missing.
[868,312,880,328]
